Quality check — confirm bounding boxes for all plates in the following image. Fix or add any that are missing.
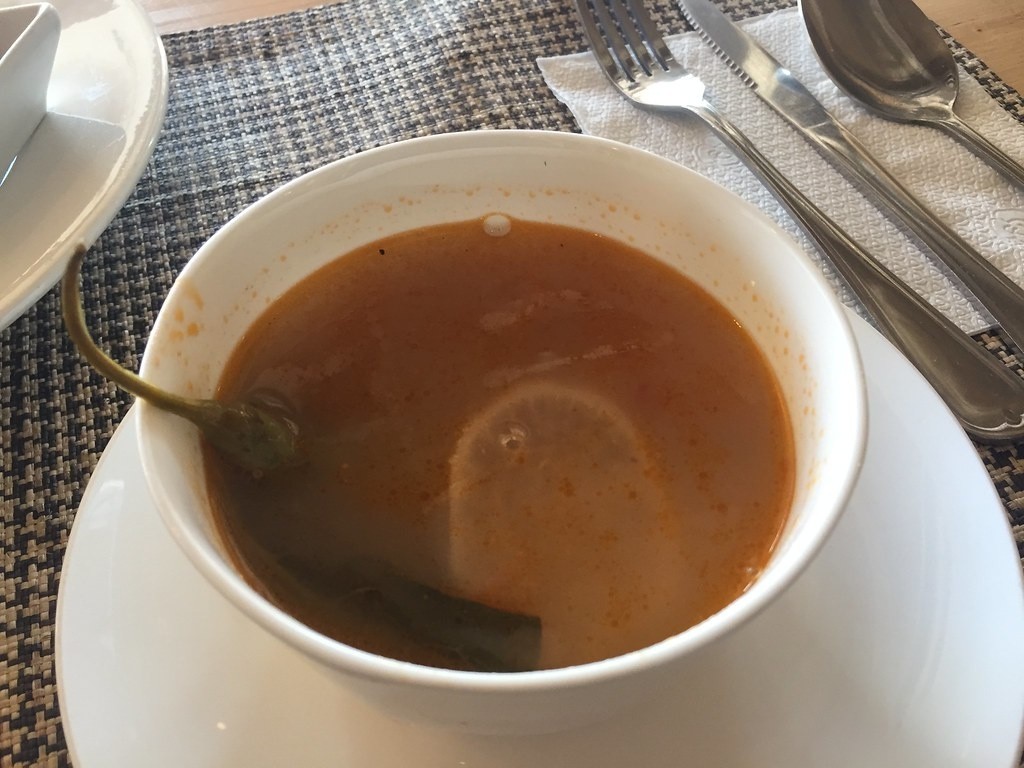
[55,303,1024,768]
[0,0,167,333]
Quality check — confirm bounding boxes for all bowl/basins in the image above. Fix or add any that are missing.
[136,130,866,694]
[0,3,59,183]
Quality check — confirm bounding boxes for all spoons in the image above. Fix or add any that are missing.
[797,0,1024,191]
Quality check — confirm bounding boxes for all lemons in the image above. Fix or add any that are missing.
[446,382,661,598]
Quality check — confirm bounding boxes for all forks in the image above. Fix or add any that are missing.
[573,0,1024,440]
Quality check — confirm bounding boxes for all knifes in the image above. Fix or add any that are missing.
[676,0,1024,354]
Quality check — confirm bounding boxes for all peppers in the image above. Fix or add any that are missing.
[364,577,543,672]
[65,242,300,487]
